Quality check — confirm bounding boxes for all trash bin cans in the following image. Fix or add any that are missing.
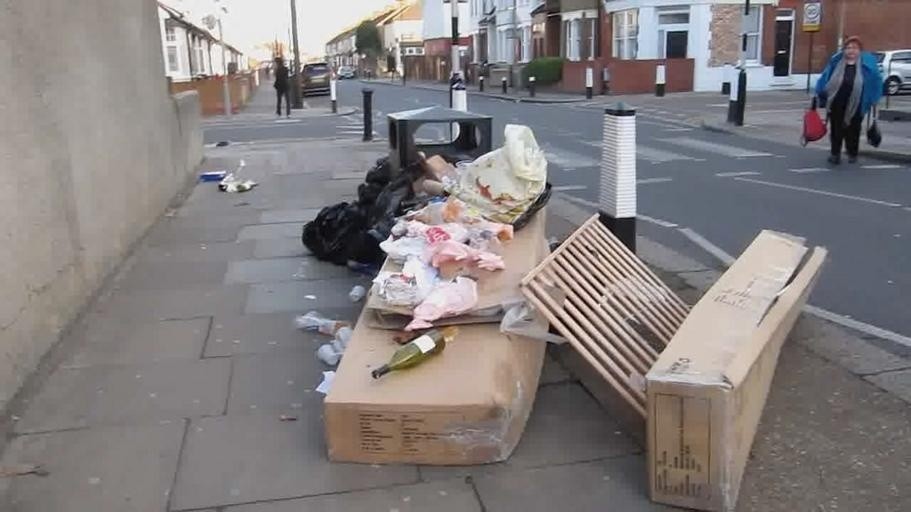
[387,103,494,181]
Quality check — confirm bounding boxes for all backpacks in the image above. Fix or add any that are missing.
[801,97,828,147]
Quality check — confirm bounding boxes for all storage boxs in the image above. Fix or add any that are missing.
[323,203,553,466]
[644,228,829,512]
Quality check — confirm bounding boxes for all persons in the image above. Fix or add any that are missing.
[272,57,291,118]
[815,35,883,164]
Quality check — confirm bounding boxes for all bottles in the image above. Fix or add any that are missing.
[371,329,445,381]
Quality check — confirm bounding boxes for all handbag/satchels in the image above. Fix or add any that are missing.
[867,119,882,147]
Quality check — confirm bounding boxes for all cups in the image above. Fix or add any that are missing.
[318,344,338,365]
[346,284,365,303]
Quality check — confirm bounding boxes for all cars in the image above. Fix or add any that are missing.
[337,65,356,81]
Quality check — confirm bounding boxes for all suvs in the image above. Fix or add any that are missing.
[300,61,338,97]
[872,48,911,96]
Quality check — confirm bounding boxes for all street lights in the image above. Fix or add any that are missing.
[217,4,234,115]
